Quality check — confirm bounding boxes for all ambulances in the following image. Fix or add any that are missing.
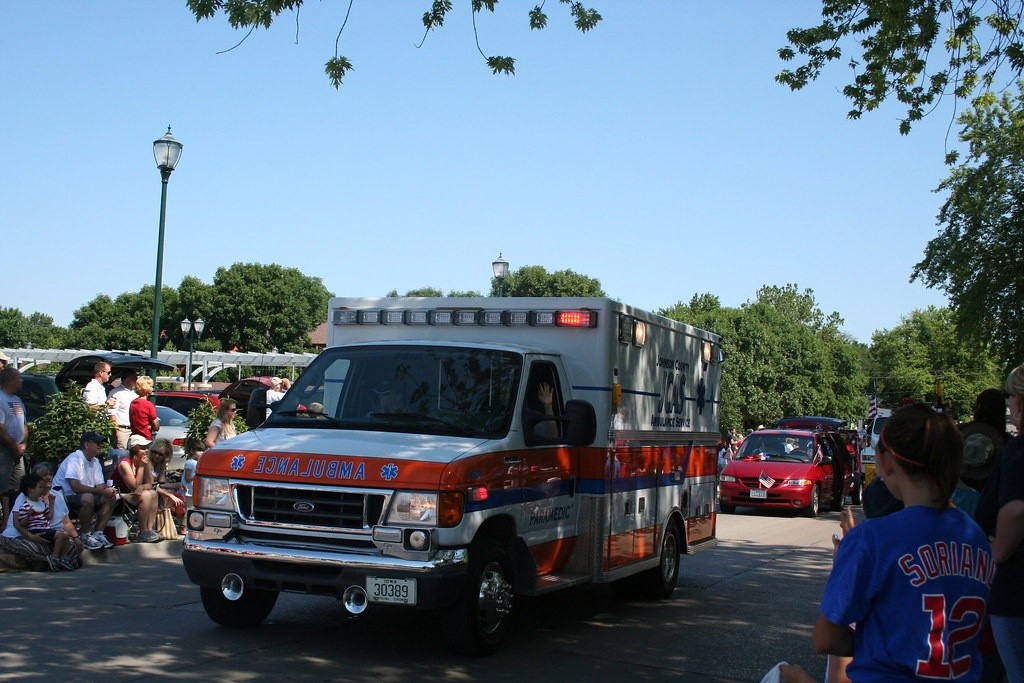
[179,294,726,658]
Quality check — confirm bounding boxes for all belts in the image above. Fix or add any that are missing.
[118,425,131,429]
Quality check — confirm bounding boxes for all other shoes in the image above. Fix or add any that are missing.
[174,517,187,535]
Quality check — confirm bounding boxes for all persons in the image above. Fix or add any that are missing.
[206,400,237,448]
[0,432,182,573]
[484,378,558,439]
[717,424,767,478]
[778,359,1024,682]
[0,349,30,532]
[82,363,161,449]
[245,377,325,431]
[178,440,206,534]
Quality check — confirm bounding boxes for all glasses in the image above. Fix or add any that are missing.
[227,408,235,412]
[153,450,164,457]
[104,371,112,375]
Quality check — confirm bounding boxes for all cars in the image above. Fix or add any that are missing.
[719,416,864,517]
[217,377,271,422]
[148,388,224,431]
[15,353,178,433]
[148,405,200,482]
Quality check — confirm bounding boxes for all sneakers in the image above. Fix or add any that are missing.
[47,554,60,571]
[59,559,74,570]
[137,532,160,543]
[92,534,114,548]
[80,535,103,549]
[149,530,165,540]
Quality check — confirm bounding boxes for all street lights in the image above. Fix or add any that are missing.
[491,252,510,297]
[144,122,184,405]
[180,315,206,390]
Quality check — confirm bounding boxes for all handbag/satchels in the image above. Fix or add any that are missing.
[152,508,179,539]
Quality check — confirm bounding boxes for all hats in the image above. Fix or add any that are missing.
[271,377,283,387]
[80,432,106,444]
[127,434,152,449]
[758,425,766,430]
[0,351,10,361]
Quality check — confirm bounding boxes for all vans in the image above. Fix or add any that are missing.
[860,408,895,501]
